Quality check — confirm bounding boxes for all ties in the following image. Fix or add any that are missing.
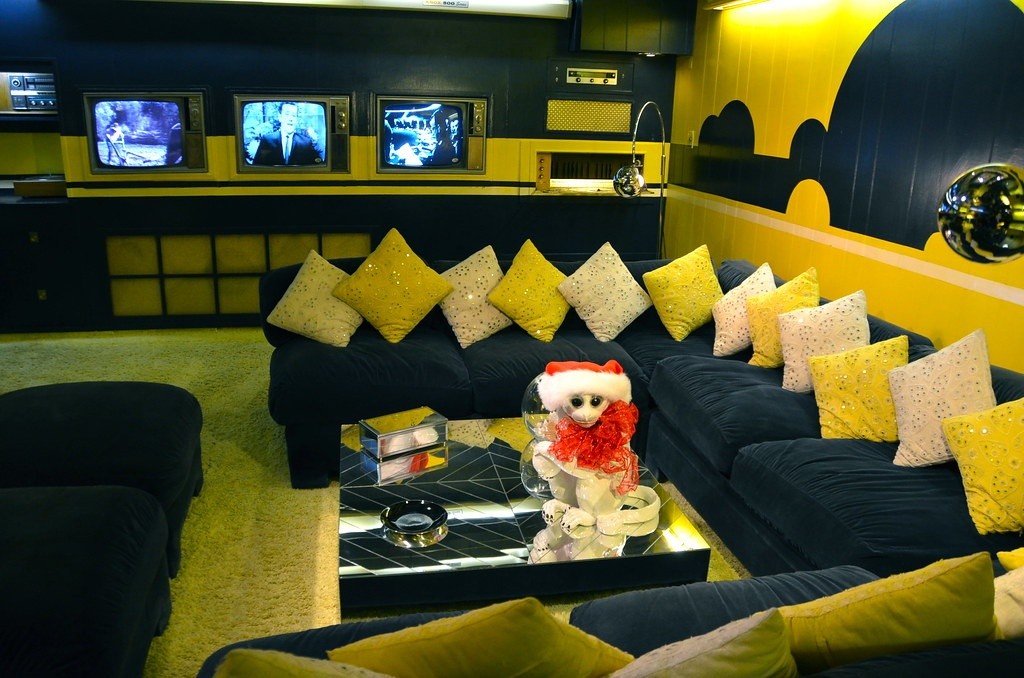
[285,135,290,163]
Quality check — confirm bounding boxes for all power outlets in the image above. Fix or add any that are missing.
[687,130,694,147]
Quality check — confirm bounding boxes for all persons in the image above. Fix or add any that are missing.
[384,110,458,166]
[105,122,127,165]
[252,102,323,166]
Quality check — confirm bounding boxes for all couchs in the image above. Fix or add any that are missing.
[211,257,948,678]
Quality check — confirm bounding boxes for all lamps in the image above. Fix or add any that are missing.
[614,100,666,257]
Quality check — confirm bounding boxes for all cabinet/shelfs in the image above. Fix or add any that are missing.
[574,0,698,55]
[0,222,110,332]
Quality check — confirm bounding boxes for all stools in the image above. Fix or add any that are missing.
[1,380,207,678]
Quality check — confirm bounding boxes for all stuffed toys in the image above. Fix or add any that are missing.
[532,360,661,531]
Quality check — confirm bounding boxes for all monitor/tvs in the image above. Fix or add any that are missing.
[234,94,351,174]
[83,92,208,175]
[377,96,488,175]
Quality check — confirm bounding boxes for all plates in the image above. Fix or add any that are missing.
[379,500,448,534]
[379,522,449,548]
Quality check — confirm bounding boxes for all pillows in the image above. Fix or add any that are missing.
[339,233,450,343]
[889,326,996,466]
[215,649,374,678]
[805,336,914,441]
[783,548,998,649]
[640,246,720,340]
[713,263,776,356]
[746,269,822,367]
[432,246,513,348]
[943,398,1024,534]
[558,240,651,340]
[598,608,800,678]
[779,291,869,392]
[326,599,632,678]
[487,241,569,345]
[267,247,360,346]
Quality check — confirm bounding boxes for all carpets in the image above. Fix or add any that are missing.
[1,327,319,678]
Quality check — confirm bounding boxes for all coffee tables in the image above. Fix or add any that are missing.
[340,414,711,617]
